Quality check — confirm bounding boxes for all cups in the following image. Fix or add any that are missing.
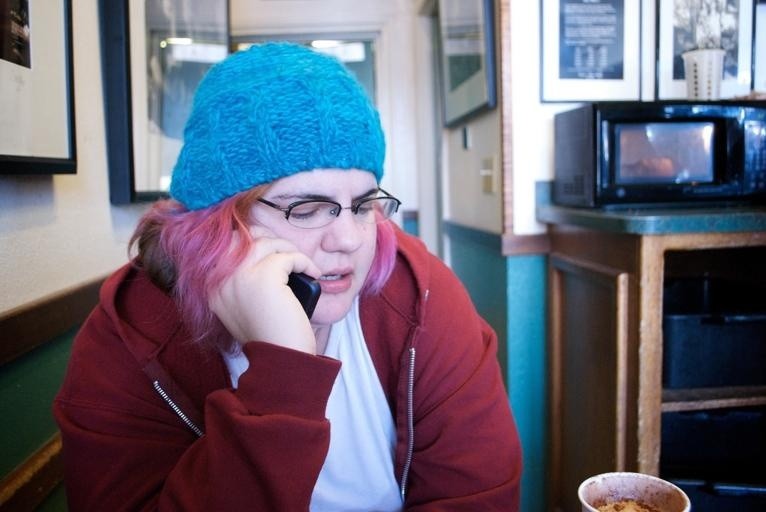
[681,47,728,103]
[576,469,692,512]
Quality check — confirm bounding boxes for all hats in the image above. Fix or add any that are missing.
[170,40,386,211]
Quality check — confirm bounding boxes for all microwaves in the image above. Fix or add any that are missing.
[552,98,766,205]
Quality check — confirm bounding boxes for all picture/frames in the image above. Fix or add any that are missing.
[93,2,231,207]
[538,0,766,104]
[0,0,78,176]
[432,0,498,129]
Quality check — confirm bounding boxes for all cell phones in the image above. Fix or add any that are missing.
[286,273,321,324]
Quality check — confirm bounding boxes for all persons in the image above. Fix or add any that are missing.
[55,30,526,511]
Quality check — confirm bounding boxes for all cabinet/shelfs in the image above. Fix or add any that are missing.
[539,226,765,512]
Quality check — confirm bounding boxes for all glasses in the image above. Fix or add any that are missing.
[256,189,401,229]
